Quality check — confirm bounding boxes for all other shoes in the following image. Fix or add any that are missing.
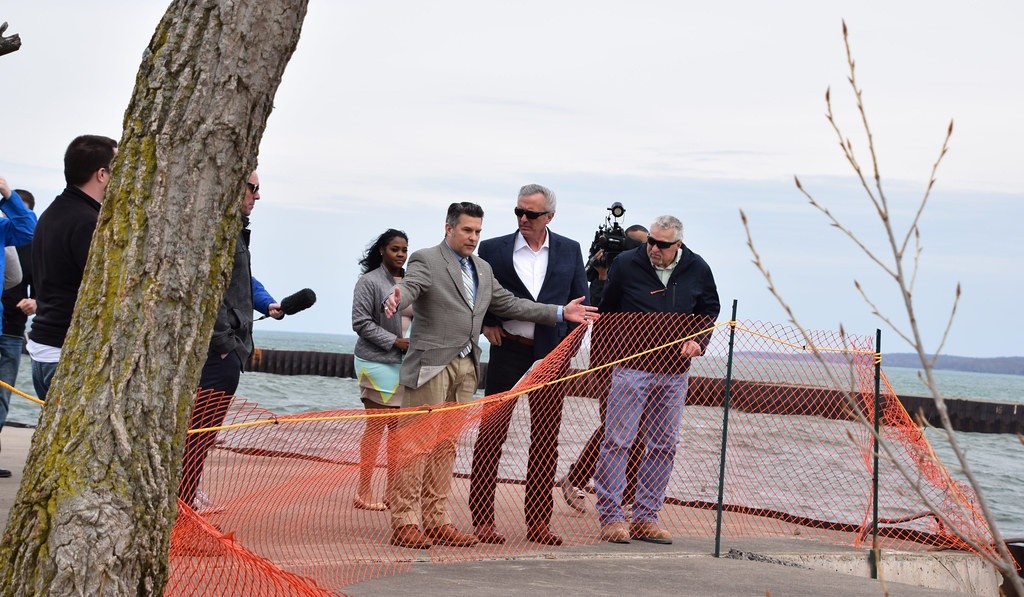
[601,522,630,543]
[382,494,393,508]
[527,525,562,546]
[623,504,634,522]
[0,470,11,477]
[190,497,214,516]
[474,524,505,544]
[562,475,585,513]
[629,522,673,543]
[196,490,226,512]
[354,489,387,511]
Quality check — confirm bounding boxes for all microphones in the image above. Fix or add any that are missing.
[259,288,317,322]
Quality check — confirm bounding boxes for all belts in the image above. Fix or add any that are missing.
[503,329,534,346]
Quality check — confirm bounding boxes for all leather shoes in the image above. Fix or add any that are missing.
[424,522,479,546]
[390,524,431,549]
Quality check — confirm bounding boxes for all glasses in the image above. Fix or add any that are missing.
[647,234,679,248]
[247,182,259,194]
[515,207,548,219]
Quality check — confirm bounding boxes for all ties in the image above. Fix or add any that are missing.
[460,258,473,359]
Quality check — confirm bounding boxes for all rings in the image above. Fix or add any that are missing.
[385,307,388,311]
[585,316,586,319]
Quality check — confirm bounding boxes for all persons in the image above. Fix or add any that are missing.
[352,230,414,510]
[559,224,651,517]
[170,170,260,556]
[26,135,118,410]
[593,216,720,543]
[469,184,589,545]
[0,176,38,477]
[381,202,600,549]
[252,275,285,321]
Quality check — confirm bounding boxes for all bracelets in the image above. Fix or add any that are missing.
[562,306,564,321]
